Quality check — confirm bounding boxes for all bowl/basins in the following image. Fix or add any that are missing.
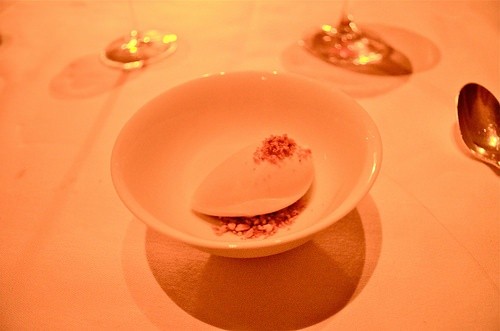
[108,69,383,259]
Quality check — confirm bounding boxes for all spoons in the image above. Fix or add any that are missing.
[457,83,500,171]
